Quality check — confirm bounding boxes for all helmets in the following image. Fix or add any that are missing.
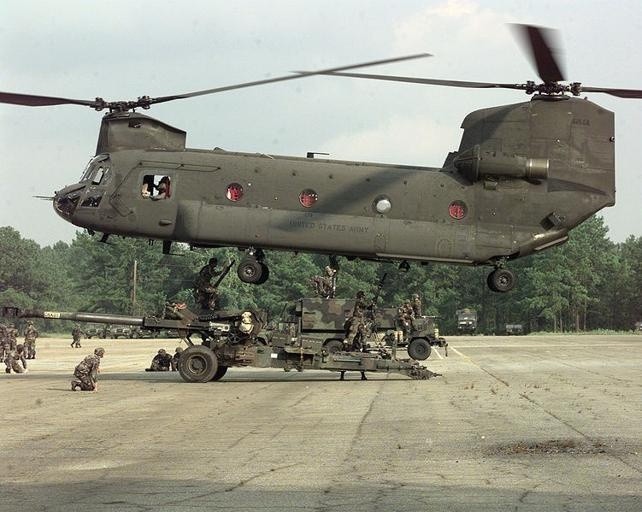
[156,184,166,191]
[158,349,166,356]
[27,321,33,325]
[175,347,183,352]
[357,291,365,298]
[209,258,218,264]
[95,347,104,356]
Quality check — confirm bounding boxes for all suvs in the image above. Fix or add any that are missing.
[505,324,524,335]
[83,321,183,338]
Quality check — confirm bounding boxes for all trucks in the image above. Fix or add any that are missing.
[278,297,448,360]
[456,308,478,336]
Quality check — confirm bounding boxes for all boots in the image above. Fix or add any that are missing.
[346,345,352,352]
[362,345,369,352]
[32,355,35,359]
[27,355,31,359]
[71,381,81,390]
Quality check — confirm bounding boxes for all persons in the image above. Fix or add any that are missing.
[150,177,170,200]
[78,324,83,347]
[195,257,221,310]
[4,322,19,357]
[146,349,170,371]
[71,322,80,348]
[4,344,28,374]
[0,324,6,363]
[172,347,183,372]
[404,299,414,322]
[23,321,38,359]
[71,347,105,391]
[345,291,374,353]
[413,293,421,316]
[325,265,336,298]
[396,308,407,341]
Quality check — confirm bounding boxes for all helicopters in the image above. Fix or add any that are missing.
[1,24,642,293]
